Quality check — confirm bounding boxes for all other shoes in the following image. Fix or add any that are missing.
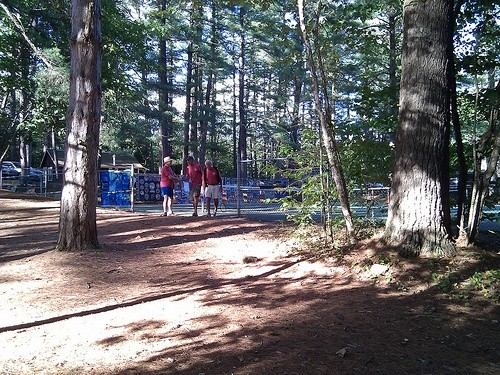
[163,212,167,216]
[211,212,216,218]
[167,214,177,216]
[205,214,211,218]
[192,213,198,217]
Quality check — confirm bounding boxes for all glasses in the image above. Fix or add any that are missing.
[168,160,172,162]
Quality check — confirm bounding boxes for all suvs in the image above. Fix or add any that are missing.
[2,162,33,175]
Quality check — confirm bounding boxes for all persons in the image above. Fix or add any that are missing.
[204,160,222,218]
[160,156,182,217]
[185,156,204,217]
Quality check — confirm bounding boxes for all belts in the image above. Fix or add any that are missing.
[209,184,218,185]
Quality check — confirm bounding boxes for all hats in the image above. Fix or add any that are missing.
[163,156,173,164]
